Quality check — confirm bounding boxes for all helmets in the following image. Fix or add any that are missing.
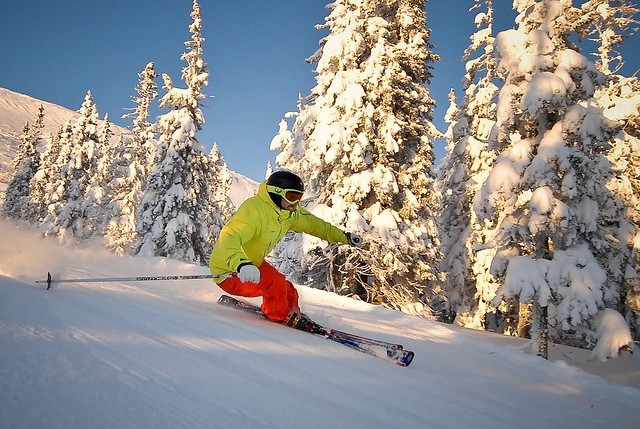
[265,170,305,210]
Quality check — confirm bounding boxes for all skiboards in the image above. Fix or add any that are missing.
[216,295,415,368]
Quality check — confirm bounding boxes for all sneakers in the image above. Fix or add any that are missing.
[283,311,320,333]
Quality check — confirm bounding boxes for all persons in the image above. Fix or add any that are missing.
[208,172,361,333]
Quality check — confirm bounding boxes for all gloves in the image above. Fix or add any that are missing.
[350,233,362,247]
[239,264,261,284]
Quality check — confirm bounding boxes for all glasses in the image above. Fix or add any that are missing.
[267,185,309,206]
